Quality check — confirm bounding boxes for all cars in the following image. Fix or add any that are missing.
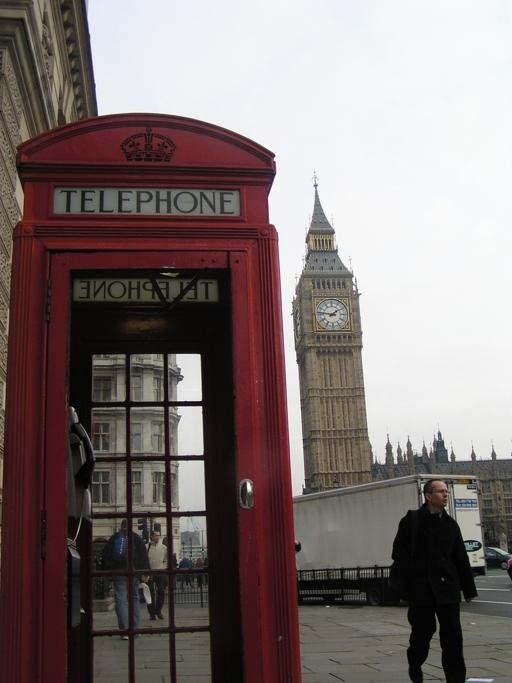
[483,547,512,582]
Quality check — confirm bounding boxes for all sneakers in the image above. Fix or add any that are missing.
[119,613,164,640]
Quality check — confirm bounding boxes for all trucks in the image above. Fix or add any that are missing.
[293,474,486,607]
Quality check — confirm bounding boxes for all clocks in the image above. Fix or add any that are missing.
[316,298,349,331]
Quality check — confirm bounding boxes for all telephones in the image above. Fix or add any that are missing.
[67,406,96,518]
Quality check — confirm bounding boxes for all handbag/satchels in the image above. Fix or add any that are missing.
[138,583,151,605]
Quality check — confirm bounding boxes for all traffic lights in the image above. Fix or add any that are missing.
[137,518,146,531]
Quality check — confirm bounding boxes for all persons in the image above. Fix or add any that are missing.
[101,520,208,639]
[388,478,479,683]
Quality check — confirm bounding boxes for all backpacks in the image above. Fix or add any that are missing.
[112,531,128,565]
